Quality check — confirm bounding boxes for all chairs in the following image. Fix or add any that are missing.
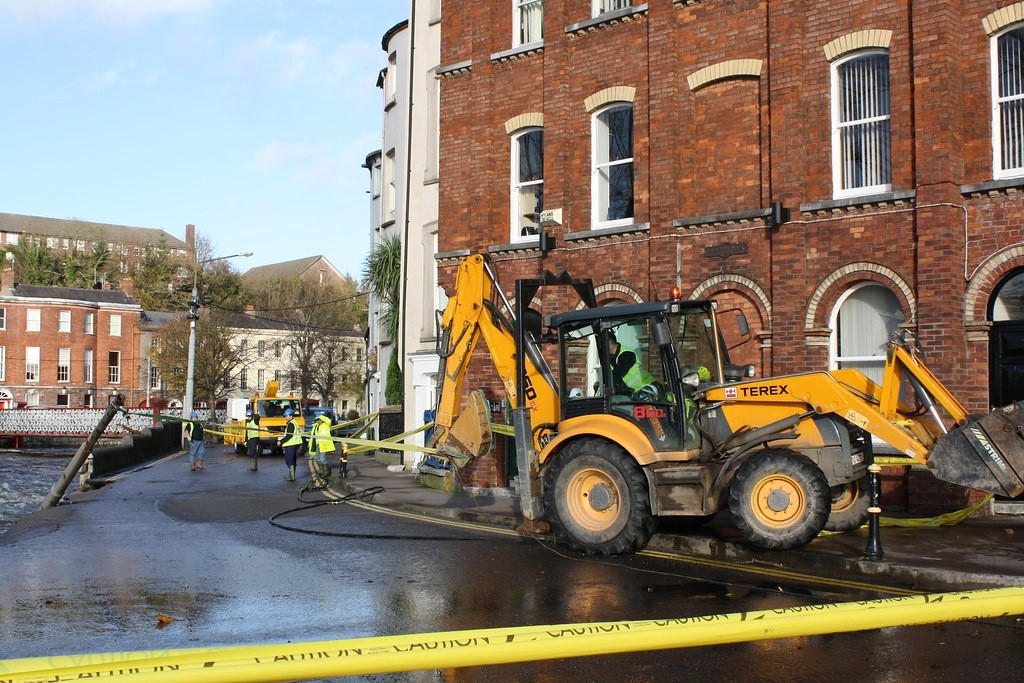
[612,370,634,397]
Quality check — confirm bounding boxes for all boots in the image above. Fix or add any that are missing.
[318,464,324,475]
[248,457,257,471]
[323,463,329,476]
[283,469,290,479]
[286,465,295,481]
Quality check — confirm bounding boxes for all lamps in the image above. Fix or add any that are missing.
[539,232,556,251]
[773,201,790,225]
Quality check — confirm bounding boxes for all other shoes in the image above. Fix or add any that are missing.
[196,460,203,469]
[191,463,196,471]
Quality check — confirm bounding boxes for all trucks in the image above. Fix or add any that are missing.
[297,406,362,451]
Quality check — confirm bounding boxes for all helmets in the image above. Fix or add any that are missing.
[246,410,253,415]
[283,409,294,416]
[191,413,198,417]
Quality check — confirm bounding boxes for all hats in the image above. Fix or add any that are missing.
[314,413,322,420]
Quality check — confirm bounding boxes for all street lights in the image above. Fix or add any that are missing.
[181,249,258,449]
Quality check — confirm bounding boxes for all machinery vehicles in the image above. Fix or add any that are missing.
[222,379,308,456]
[418,251,1024,558]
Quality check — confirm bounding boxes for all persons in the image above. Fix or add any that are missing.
[185,411,206,471]
[593,330,711,450]
[245,399,345,480]
